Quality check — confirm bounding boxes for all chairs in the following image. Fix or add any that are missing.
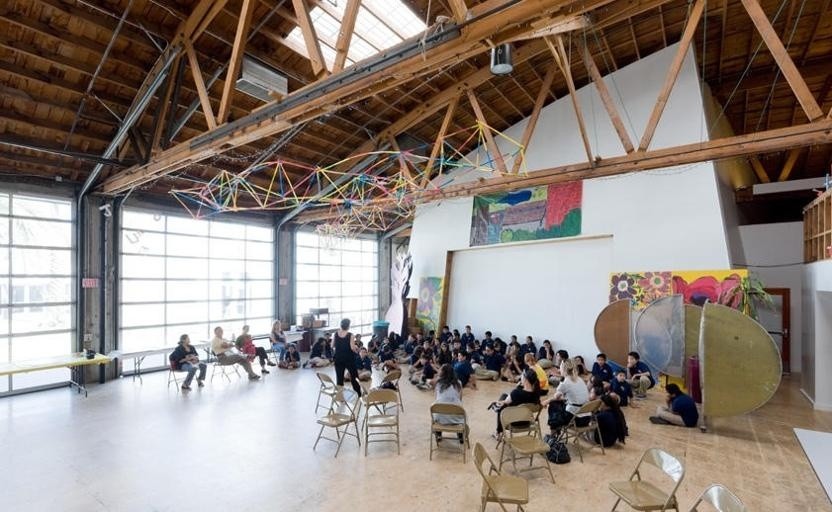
[429,402,470,464]
[499,406,557,484]
[607,447,687,512]
[208,348,241,382]
[365,389,400,457]
[555,398,606,464]
[517,403,543,441]
[688,482,746,512]
[314,371,347,415]
[472,441,530,512]
[313,388,361,458]
[167,351,199,392]
[268,337,284,366]
[371,370,405,415]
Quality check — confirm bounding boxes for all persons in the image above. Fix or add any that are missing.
[354,334,363,348]
[538,340,555,369]
[574,356,587,378]
[519,336,537,370]
[590,353,613,392]
[269,320,289,369]
[624,352,656,398]
[330,319,368,405]
[545,350,569,386]
[475,343,506,381]
[649,384,699,428]
[283,343,302,370]
[302,337,330,369]
[583,395,626,447]
[494,337,507,354]
[343,348,372,381]
[609,392,629,437]
[607,370,641,408]
[492,368,541,443]
[170,334,207,390]
[515,353,549,396]
[480,330,494,361]
[432,363,470,445]
[380,326,479,388]
[507,334,521,360]
[234,325,276,374]
[324,333,335,361]
[210,327,261,380]
[368,334,383,363]
[539,359,592,439]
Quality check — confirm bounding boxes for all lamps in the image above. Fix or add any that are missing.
[489,43,514,75]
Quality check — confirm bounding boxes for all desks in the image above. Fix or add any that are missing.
[251,330,303,343]
[109,340,213,384]
[0,351,111,398]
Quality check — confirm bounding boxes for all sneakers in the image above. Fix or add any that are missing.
[267,361,276,367]
[197,382,205,387]
[261,369,270,374]
[248,373,261,380]
[182,385,192,392]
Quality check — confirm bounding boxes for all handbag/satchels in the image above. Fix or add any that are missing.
[547,442,571,465]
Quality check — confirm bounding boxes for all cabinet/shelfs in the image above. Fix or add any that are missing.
[802,187,832,264]
